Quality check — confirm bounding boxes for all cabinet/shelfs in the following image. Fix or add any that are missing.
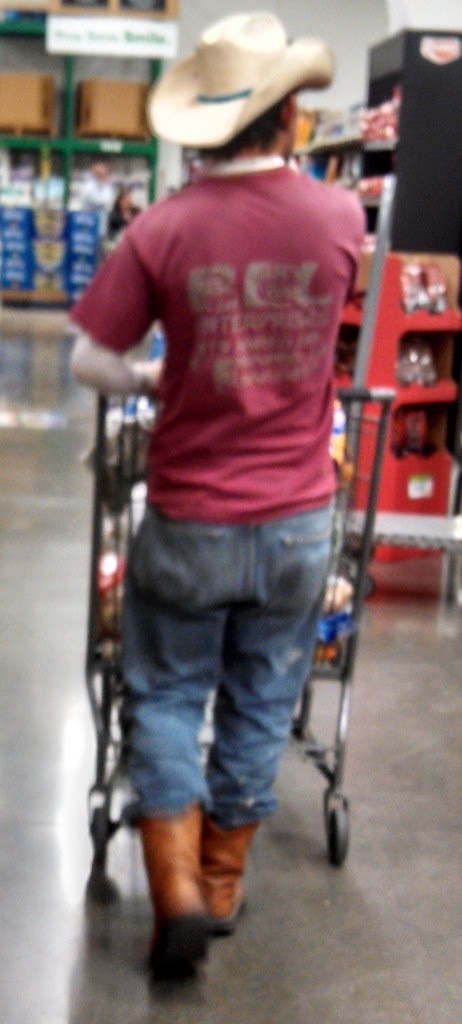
[0,0,182,310]
[293,27,462,600]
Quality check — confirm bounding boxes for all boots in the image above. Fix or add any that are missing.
[131,802,258,974]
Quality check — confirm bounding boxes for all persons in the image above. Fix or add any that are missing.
[63,9,369,1001]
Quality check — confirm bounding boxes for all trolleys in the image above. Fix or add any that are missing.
[78,376,395,908]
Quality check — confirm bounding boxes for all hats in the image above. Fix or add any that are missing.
[145,11,332,150]
